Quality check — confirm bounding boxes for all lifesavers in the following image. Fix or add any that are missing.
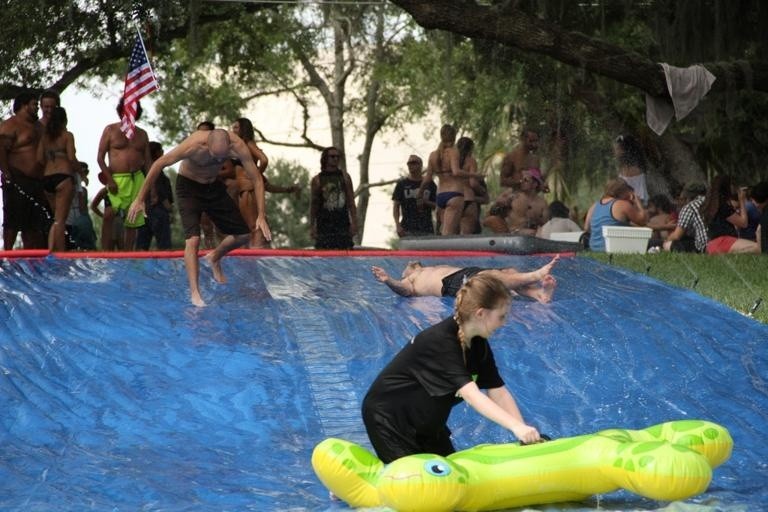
[311,419,735,512]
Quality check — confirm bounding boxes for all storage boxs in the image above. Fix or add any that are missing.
[601,225,653,255]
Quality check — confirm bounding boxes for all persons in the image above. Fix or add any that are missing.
[363,275,541,467]
[584,133,768,254]
[309,147,360,251]
[0,90,301,308]
[483,131,583,244]
[391,124,489,239]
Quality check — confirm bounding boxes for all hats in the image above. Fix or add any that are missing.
[522,169,542,183]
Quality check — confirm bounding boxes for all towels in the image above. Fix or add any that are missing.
[645,59,716,135]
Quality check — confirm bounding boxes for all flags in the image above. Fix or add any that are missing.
[120,33,160,142]
